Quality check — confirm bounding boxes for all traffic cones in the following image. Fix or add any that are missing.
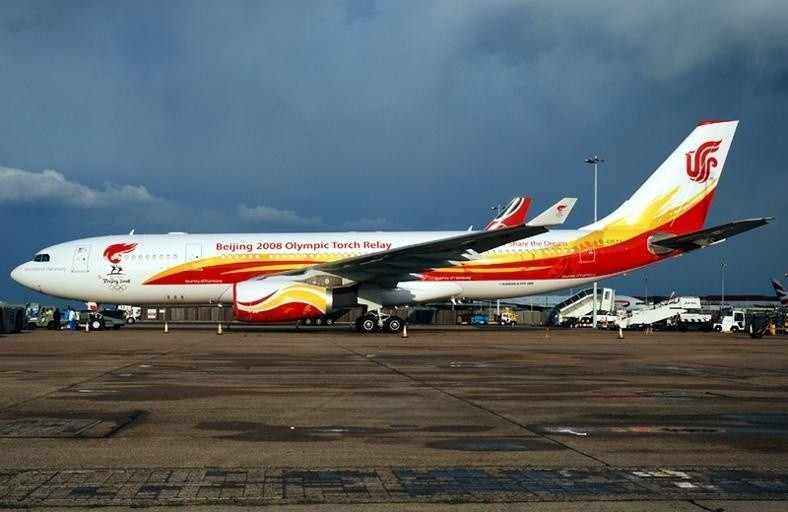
[85,322,90,332]
[164,322,168,333]
[216,324,223,334]
[402,324,408,338]
[618,328,624,339]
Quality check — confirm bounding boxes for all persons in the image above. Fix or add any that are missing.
[69,308,76,331]
[64,305,71,329]
[53,308,64,330]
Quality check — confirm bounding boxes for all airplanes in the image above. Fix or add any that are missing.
[10,119,776,332]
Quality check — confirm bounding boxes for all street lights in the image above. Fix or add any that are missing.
[583,155,604,329]
